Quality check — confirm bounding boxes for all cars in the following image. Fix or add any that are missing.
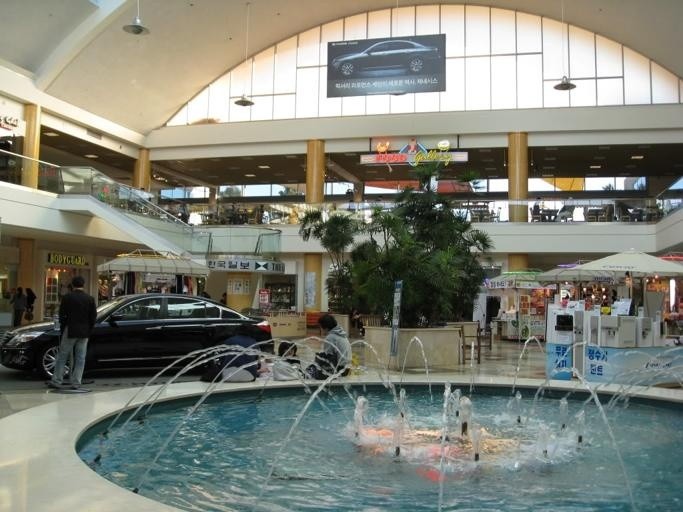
[332,40,444,79]
[0,293,274,382]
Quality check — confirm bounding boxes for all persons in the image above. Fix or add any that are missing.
[318,315,352,376]
[26,288,36,324]
[534,197,546,222]
[350,308,365,337]
[210,322,261,383]
[97,279,110,301]
[278,342,306,379]
[10,286,26,327]
[45,276,97,390]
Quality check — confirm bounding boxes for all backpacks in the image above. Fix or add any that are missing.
[200,346,222,382]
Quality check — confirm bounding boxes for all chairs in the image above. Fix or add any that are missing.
[467,204,502,221]
[528,205,573,222]
[444,321,480,363]
[582,204,663,222]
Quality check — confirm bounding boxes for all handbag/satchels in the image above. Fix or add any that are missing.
[315,352,337,380]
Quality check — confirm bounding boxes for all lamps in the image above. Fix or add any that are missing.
[232,2,253,105]
[121,2,149,36]
[551,0,575,91]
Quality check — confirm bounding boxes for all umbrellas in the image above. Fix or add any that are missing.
[483,248,683,310]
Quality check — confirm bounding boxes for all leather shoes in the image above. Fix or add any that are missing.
[46,380,81,389]
